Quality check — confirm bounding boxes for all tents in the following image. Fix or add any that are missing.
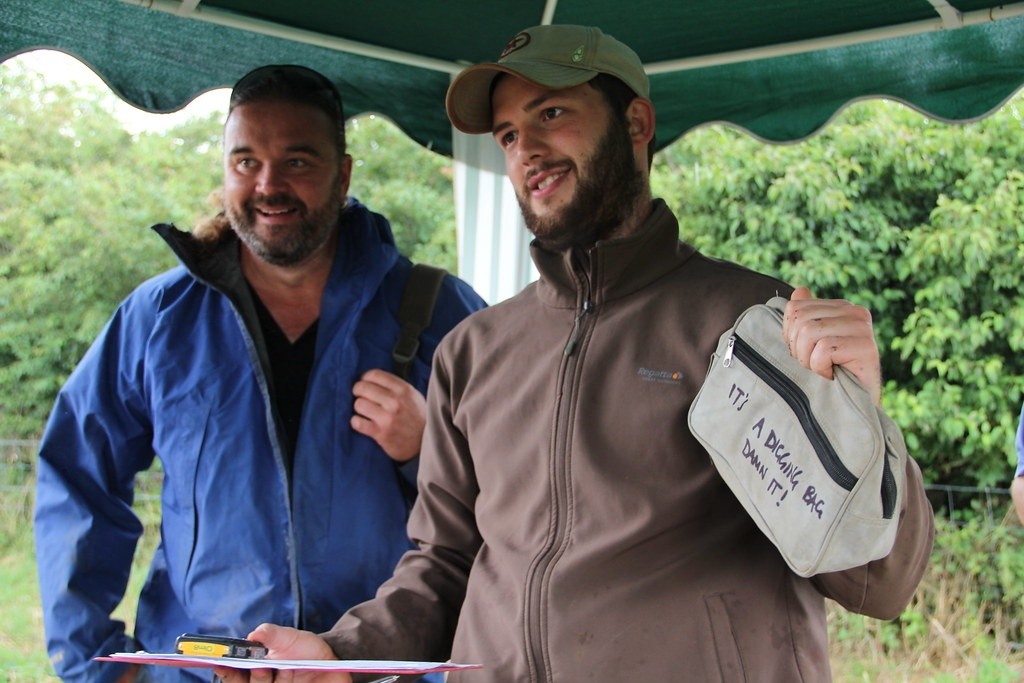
[0,0,1024,306]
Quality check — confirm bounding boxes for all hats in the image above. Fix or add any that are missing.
[447,22,651,135]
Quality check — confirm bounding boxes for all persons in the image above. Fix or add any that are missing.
[214,22,936,683]
[1009,401,1024,523]
[35,64,491,683]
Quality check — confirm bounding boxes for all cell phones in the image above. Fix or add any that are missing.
[174,633,266,661]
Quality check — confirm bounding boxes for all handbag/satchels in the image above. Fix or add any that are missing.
[685,295,904,578]
